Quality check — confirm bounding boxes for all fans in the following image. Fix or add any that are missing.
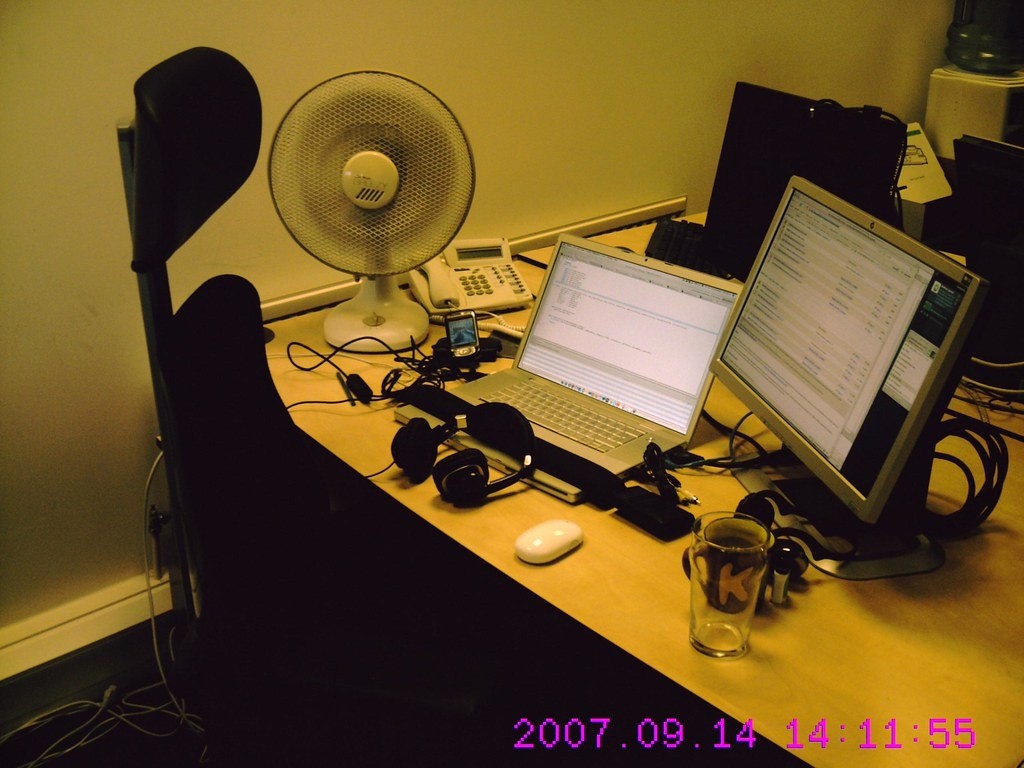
[271,70,475,353]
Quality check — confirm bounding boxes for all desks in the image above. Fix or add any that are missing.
[264,265,1024,768]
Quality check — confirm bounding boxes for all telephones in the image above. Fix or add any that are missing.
[405,236,537,325]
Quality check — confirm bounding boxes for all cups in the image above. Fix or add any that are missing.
[682,517,775,615]
[688,511,771,659]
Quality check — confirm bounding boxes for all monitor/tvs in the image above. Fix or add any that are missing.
[701,80,907,285]
[707,174,992,582]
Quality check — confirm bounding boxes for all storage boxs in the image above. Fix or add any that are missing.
[897,121,963,250]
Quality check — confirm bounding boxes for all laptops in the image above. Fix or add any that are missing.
[393,233,743,507]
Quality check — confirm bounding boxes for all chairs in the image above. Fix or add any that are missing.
[118,46,699,768]
[702,81,907,282]
[953,134,1024,276]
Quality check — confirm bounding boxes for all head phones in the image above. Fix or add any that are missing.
[392,402,540,509]
[733,489,858,581]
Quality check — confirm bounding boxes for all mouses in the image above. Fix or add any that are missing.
[516,518,583,566]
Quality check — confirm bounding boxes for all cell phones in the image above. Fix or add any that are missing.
[445,309,482,363]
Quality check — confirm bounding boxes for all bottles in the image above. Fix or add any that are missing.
[944,0,1024,74]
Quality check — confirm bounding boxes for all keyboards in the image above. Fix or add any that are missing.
[645,218,728,282]
